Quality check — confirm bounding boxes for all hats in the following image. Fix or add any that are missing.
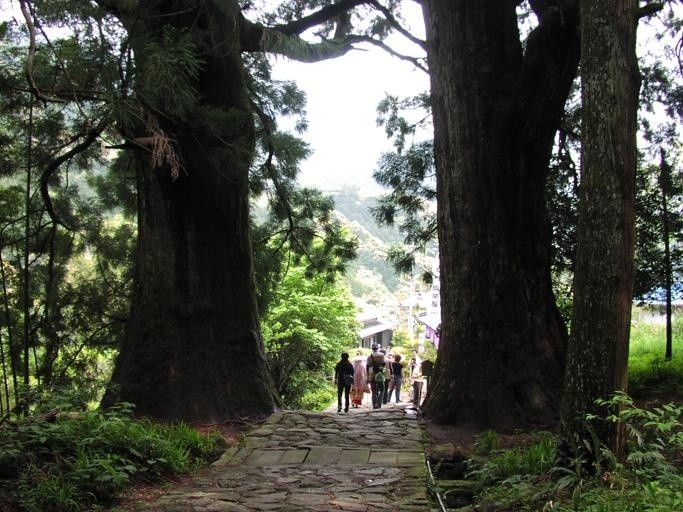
[371,343,379,350]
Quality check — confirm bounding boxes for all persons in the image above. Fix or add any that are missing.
[364,342,423,410]
[334,352,354,413]
[349,355,370,408]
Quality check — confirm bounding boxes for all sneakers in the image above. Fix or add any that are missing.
[351,402,357,407]
[336,406,349,413]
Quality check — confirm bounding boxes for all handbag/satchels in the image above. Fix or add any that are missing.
[368,364,391,381]
[343,374,355,388]
